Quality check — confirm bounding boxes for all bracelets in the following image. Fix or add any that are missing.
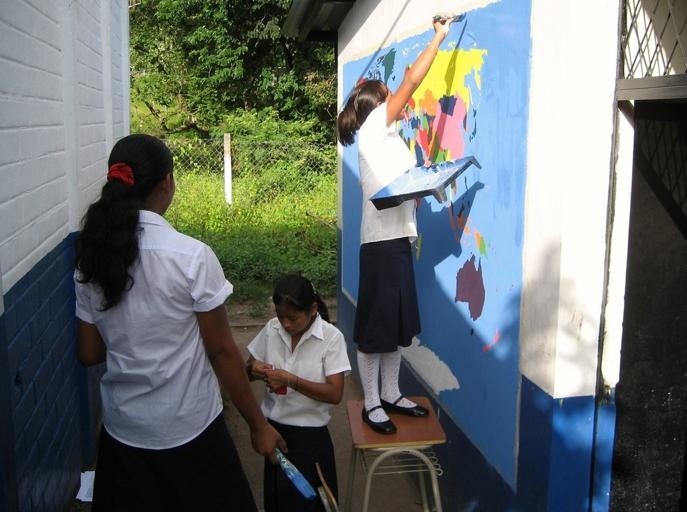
[295,374,301,392]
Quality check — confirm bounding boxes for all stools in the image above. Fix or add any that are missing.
[345,395,447,511]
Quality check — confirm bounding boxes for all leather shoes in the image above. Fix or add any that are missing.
[362,404,397,434]
[380,395,429,417]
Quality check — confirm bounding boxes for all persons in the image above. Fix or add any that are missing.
[245,273,353,512]
[72,135,288,512]
[338,12,454,434]
